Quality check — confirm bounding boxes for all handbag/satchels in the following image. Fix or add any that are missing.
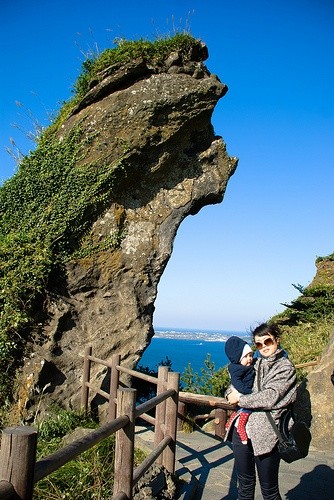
[277,410,312,464]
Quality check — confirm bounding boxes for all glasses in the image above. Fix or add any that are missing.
[255,338,274,350]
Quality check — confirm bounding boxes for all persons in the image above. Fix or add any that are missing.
[224,322,298,500]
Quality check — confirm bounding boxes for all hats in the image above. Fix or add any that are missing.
[238,343,253,362]
